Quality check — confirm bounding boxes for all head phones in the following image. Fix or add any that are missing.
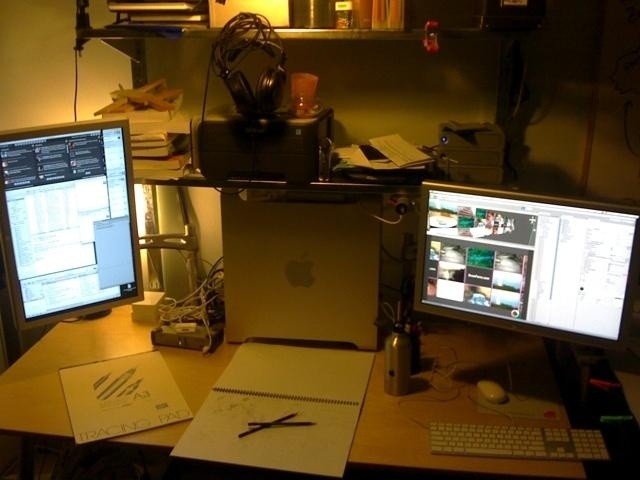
[214,13,289,113]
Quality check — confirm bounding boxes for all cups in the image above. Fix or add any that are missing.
[287,71,320,106]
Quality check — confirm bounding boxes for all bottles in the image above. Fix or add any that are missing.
[383,322,413,395]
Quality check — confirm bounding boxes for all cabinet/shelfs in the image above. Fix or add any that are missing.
[76,29,530,197]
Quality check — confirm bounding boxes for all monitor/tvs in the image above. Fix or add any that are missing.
[413,179,640,354]
[0,116,145,333]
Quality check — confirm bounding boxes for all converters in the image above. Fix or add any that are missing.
[132,290,167,323]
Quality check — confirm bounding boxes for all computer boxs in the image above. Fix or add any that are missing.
[220,187,382,350]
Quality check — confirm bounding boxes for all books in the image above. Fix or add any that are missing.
[101,93,192,171]
[107,1,209,30]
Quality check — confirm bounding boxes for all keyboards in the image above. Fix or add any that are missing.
[428,422,611,461]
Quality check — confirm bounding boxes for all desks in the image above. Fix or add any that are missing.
[0,302,587,480]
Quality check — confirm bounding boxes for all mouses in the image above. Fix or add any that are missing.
[477,378,509,404]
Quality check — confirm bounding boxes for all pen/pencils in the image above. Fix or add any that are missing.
[397,299,423,334]
[237,412,317,438]
